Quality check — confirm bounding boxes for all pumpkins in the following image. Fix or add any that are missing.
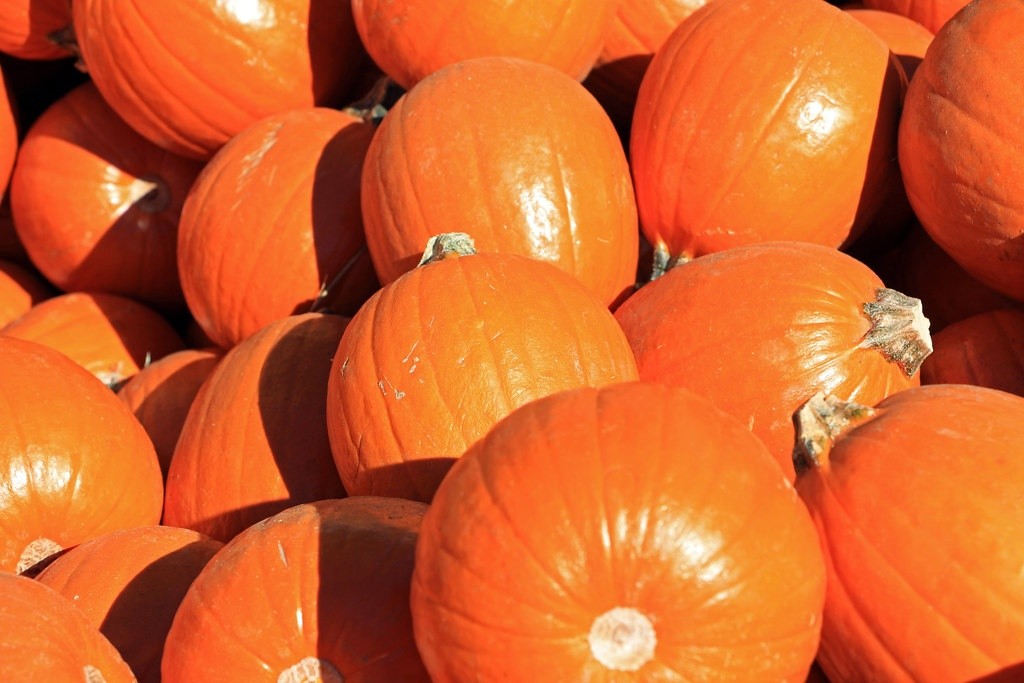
[0,0,1024,682]
